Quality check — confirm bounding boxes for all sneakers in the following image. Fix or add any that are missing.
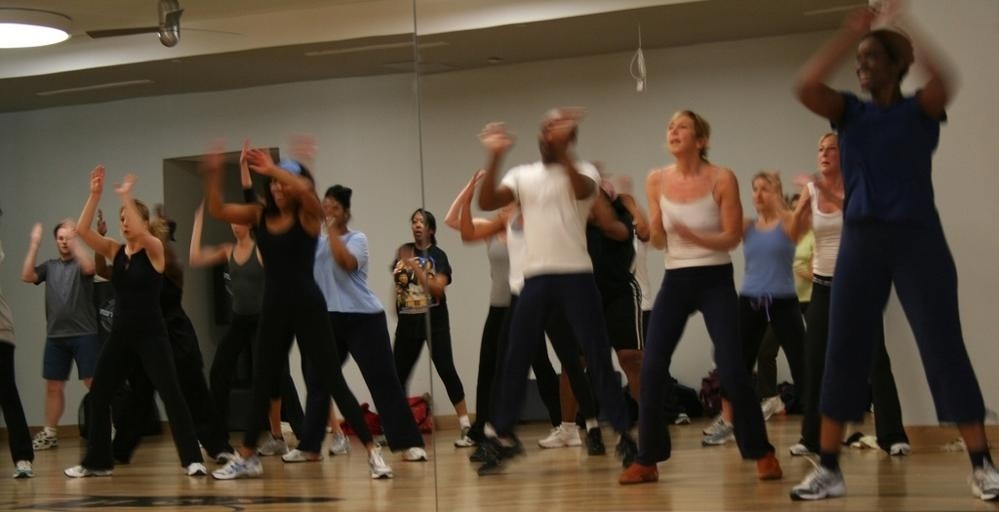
[329,433,352,456]
[210,448,264,479]
[281,449,325,462]
[615,431,637,465]
[702,421,736,446]
[619,463,658,485]
[454,435,476,447]
[32,431,60,450]
[586,427,605,456]
[462,427,471,439]
[403,447,428,461]
[761,395,784,421]
[754,455,783,480]
[13,460,36,479]
[674,412,691,424]
[65,466,112,478]
[258,436,290,455]
[703,415,725,435]
[940,438,966,453]
[967,460,999,500]
[538,422,582,448]
[789,467,845,500]
[790,444,810,455]
[891,443,909,455]
[469,441,492,461]
[187,463,207,476]
[479,439,526,475]
[216,452,234,464]
[368,448,393,478]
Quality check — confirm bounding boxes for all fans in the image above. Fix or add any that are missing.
[86,0,247,46]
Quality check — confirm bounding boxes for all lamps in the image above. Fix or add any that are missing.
[0,10,73,48]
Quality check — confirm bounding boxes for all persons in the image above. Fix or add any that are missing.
[537,184,643,449]
[790,2,998,501]
[283,186,428,463]
[200,138,395,479]
[756,193,815,416]
[617,110,784,484]
[233,135,356,463]
[781,133,909,457]
[703,173,807,446]
[383,208,468,446]
[65,166,207,477]
[21,218,97,450]
[477,106,639,476]
[0,211,37,479]
[190,205,350,460]
[462,170,560,464]
[444,169,562,445]
[112,221,232,463]
